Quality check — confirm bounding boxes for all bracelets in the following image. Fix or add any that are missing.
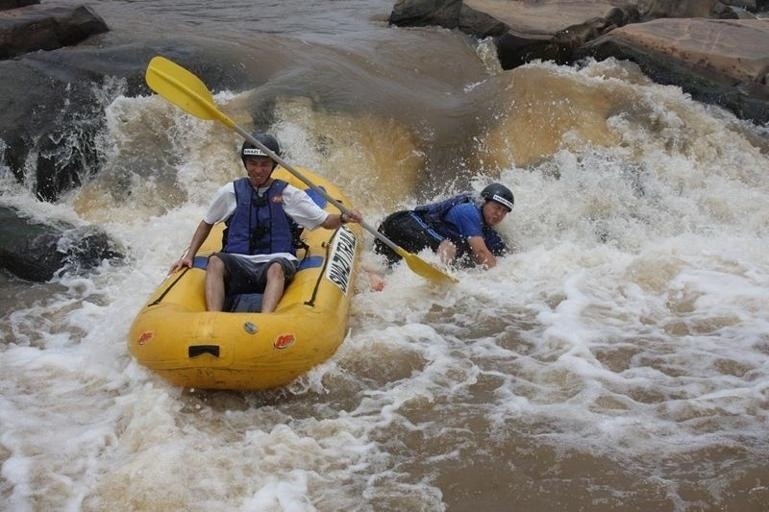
[339,212,348,225]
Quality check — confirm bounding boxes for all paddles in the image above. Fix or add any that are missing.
[146,56,459,284]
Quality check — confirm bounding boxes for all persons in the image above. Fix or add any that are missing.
[369,182,518,291]
[165,133,363,313]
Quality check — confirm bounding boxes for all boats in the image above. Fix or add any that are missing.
[124,163,363,399]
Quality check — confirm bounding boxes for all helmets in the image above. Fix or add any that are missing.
[240,132,280,160]
[479,182,515,212]
